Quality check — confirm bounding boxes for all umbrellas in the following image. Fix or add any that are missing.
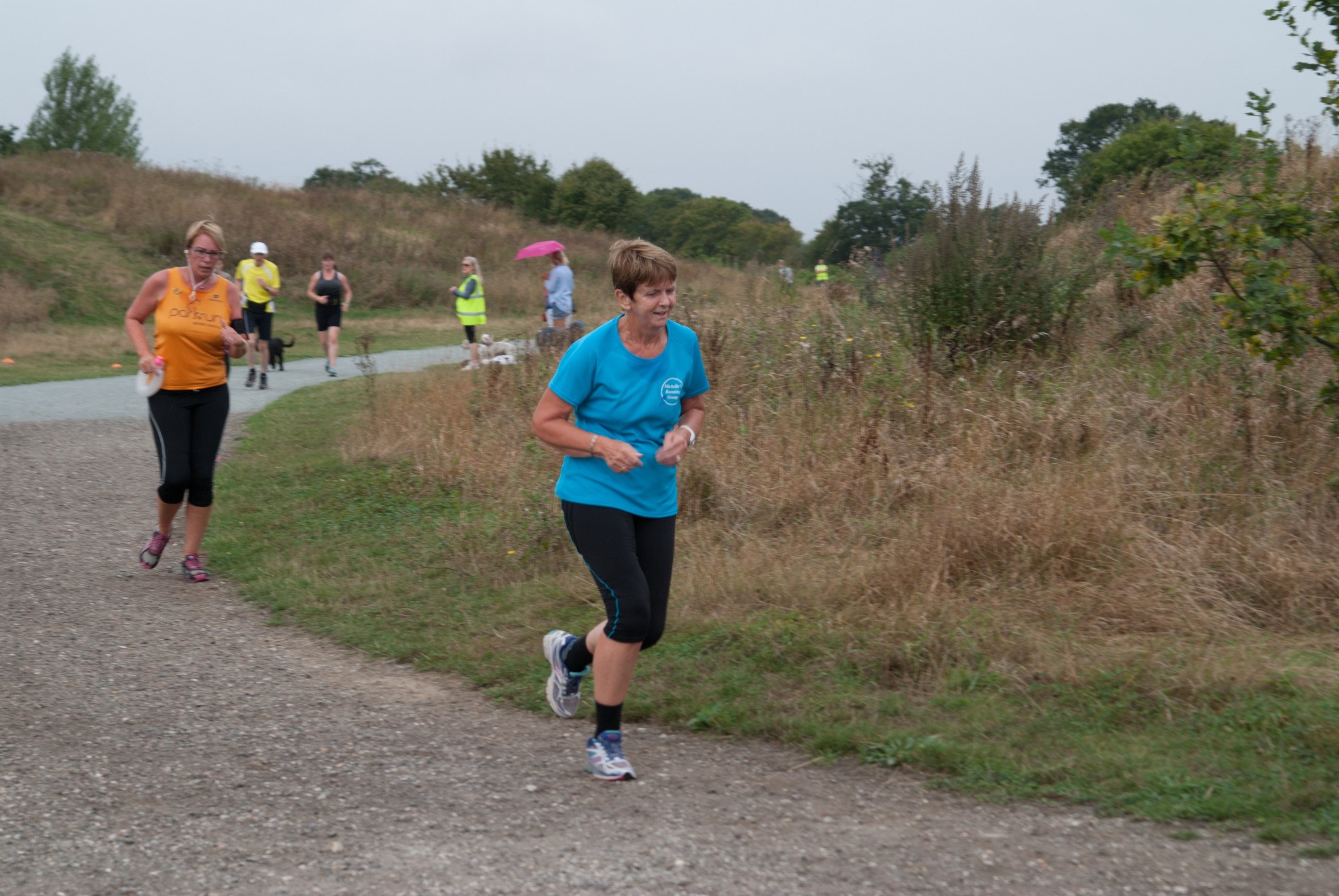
[516,241,565,260]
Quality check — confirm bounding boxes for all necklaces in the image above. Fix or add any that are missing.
[199,286,205,289]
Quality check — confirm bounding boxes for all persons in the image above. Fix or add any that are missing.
[541,250,576,329]
[124,220,248,584]
[814,259,829,287]
[214,256,234,284]
[235,242,281,389]
[777,260,793,283]
[307,253,353,376]
[449,256,486,372]
[530,238,710,781]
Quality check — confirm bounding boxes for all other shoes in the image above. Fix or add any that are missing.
[325,361,336,377]
[245,372,256,387]
[260,372,267,389]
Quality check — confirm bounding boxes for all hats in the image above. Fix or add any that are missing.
[250,242,267,255]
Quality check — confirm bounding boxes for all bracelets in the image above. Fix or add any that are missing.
[588,434,598,455]
[235,339,248,350]
[345,302,350,304]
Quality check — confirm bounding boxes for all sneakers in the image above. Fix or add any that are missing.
[543,629,590,720]
[586,730,638,781]
[138,523,172,569]
[181,554,209,582]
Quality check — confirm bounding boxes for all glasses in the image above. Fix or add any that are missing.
[461,263,472,267]
[189,244,222,259]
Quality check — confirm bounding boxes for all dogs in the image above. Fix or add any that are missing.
[255,337,295,371]
[461,333,516,357]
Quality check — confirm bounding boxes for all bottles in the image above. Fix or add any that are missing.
[135,354,166,398]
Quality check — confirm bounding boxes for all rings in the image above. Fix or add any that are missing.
[676,455,681,461]
[619,467,626,471]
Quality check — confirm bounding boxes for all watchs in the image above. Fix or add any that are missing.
[679,425,695,446]
